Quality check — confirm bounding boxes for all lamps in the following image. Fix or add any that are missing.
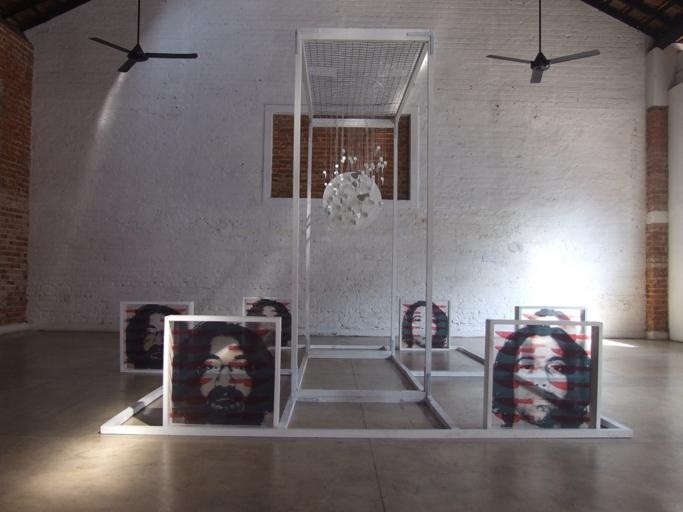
[322,81,388,230]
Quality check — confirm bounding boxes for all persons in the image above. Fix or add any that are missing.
[172,320,275,426]
[526,309,570,328]
[126,303,181,370]
[402,301,446,348]
[247,299,292,347]
[491,325,592,429]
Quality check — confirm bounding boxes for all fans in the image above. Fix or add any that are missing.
[486,0,601,84]
[88,0,198,72]
